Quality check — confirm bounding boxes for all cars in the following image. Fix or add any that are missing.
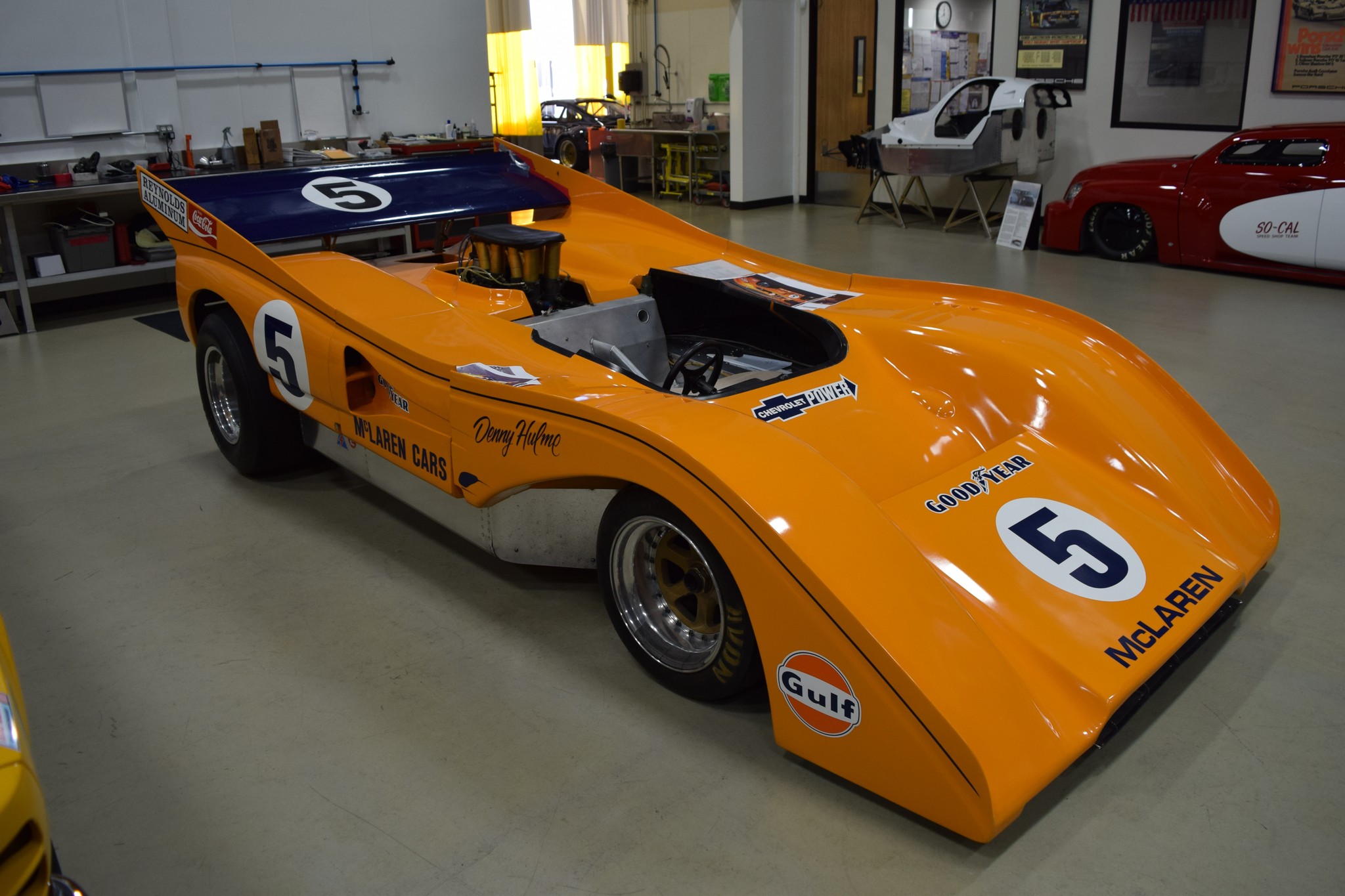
[1027,1,1079,29]
[1041,121,1344,290]
[540,98,632,171]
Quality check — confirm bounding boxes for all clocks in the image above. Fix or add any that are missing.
[936,1,952,29]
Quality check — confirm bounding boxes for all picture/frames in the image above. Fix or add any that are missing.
[1015,0,1094,90]
[1110,0,1256,132]
[1271,0,1345,95]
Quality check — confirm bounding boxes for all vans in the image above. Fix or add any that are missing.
[1010,188,1033,206]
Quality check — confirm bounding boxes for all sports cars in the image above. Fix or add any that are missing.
[129,143,1282,849]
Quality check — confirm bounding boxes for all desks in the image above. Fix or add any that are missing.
[608,121,730,206]
[0,136,501,334]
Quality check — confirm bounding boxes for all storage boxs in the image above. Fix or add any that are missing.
[43,219,116,274]
